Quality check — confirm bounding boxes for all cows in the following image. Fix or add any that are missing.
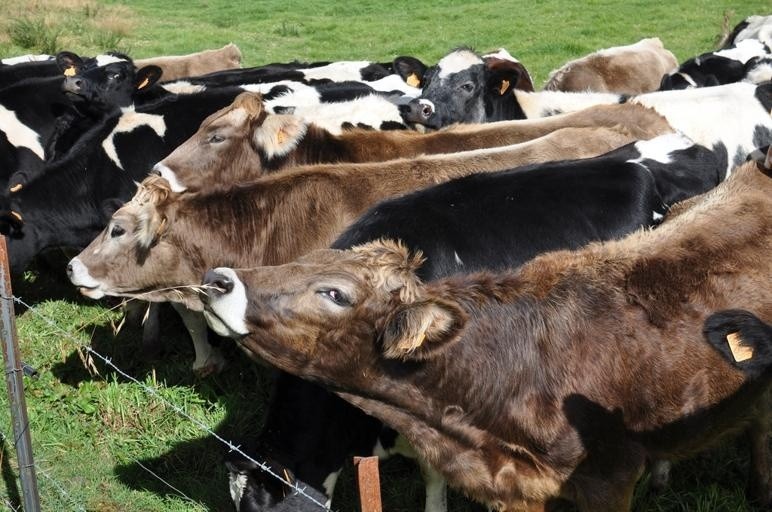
[65,124,637,312]
[0,10,771,152]
[150,92,677,191]
[198,150,771,512]
[229,137,728,512]
[0,79,403,374]
